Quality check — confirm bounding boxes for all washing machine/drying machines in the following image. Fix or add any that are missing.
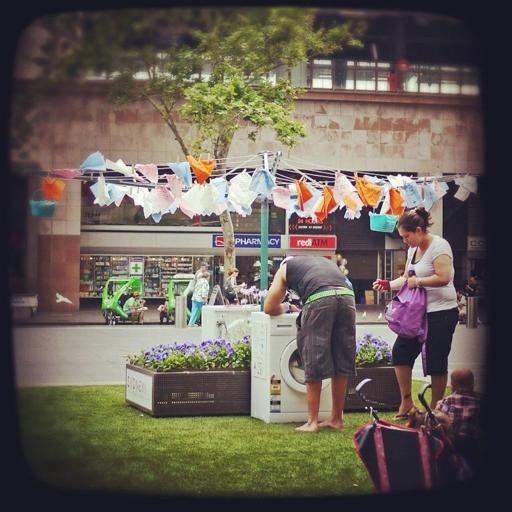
[250,311,343,424]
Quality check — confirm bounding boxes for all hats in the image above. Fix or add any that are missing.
[200,262,210,267]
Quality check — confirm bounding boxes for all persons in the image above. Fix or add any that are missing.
[121,261,251,329]
[262,251,358,435]
[406,406,468,476]
[453,272,484,325]
[433,365,486,435]
[371,203,461,423]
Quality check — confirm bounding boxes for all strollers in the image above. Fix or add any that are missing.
[349,375,478,493]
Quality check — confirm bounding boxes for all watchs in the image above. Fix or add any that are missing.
[283,300,293,313]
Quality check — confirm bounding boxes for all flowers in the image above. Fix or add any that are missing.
[122,334,252,371]
[354,333,395,365]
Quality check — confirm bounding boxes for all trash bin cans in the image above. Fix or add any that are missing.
[466,296,478,328]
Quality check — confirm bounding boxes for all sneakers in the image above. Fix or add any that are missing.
[394,404,415,421]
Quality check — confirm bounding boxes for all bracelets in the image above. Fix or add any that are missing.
[415,275,423,289]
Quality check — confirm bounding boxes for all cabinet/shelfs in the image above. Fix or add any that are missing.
[79,254,215,299]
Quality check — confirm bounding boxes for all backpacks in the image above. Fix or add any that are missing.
[384,269,429,378]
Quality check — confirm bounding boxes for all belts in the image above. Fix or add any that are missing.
[304,288,355,305]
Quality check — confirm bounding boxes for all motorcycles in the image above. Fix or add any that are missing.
[100,275,144,326]
[159,271,202,325]
